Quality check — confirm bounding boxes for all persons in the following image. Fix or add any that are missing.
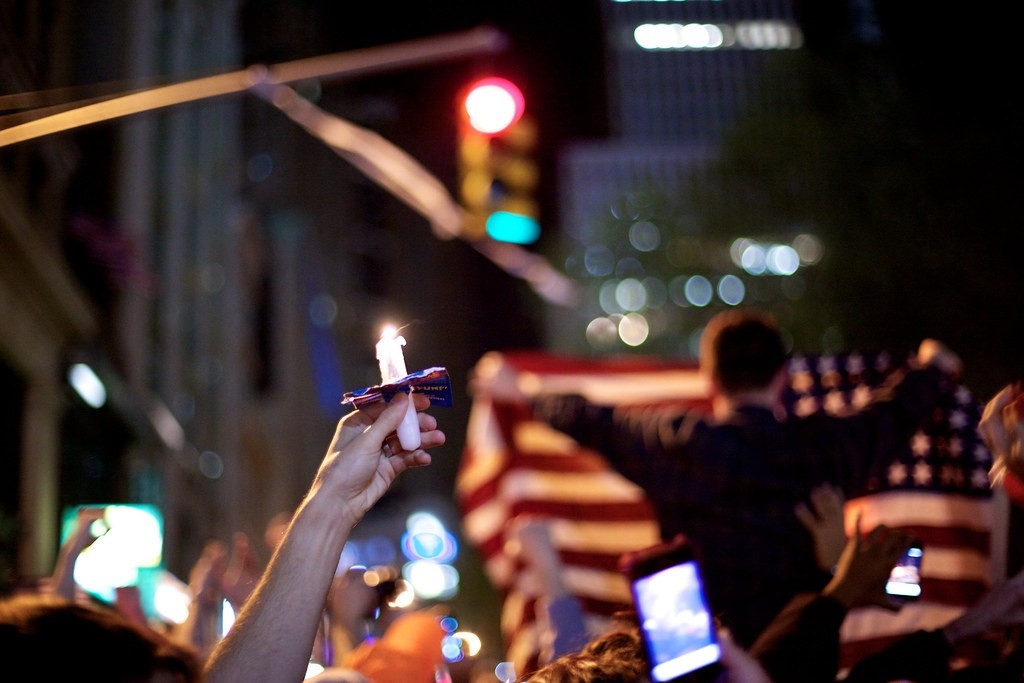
[467,308,964,655]
[514,379,1024,683]
[0,391,447,683]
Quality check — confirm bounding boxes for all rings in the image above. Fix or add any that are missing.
[382,441,393,458]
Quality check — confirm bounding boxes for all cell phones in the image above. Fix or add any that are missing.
[885,538,923,600]
[623,534,723,683]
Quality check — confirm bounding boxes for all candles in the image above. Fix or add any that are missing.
[373,322,421,451]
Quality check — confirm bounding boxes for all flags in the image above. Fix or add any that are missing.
[454,348,997,683]
